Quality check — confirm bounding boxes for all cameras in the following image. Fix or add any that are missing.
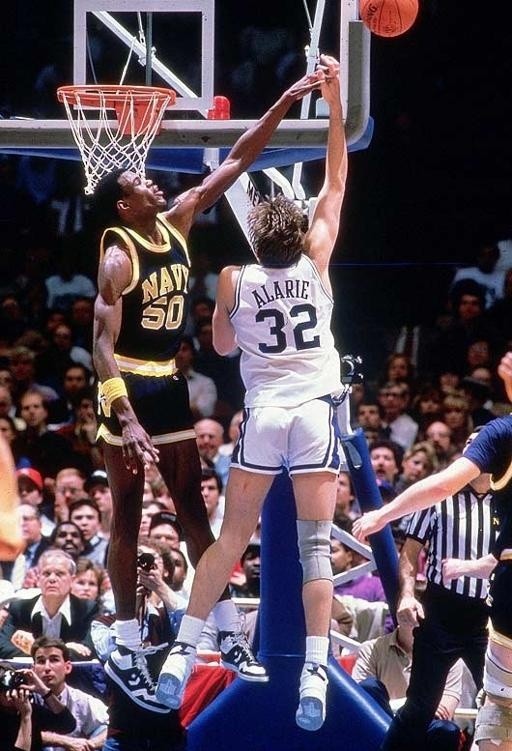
[339,353,366,387]
[136,552,156,571]
[1,668,24,692]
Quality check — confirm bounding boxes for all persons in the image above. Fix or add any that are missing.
[0,41,510,751]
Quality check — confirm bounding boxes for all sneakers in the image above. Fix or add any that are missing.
[104,647,170,713]
[156,641,193,711]
[216,629,270,682]
[296,665,328,732]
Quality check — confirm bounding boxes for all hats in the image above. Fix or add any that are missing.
[83,470,108,490]
[16,467,42,491]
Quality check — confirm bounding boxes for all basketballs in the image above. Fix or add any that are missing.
[359,0,418,38]
